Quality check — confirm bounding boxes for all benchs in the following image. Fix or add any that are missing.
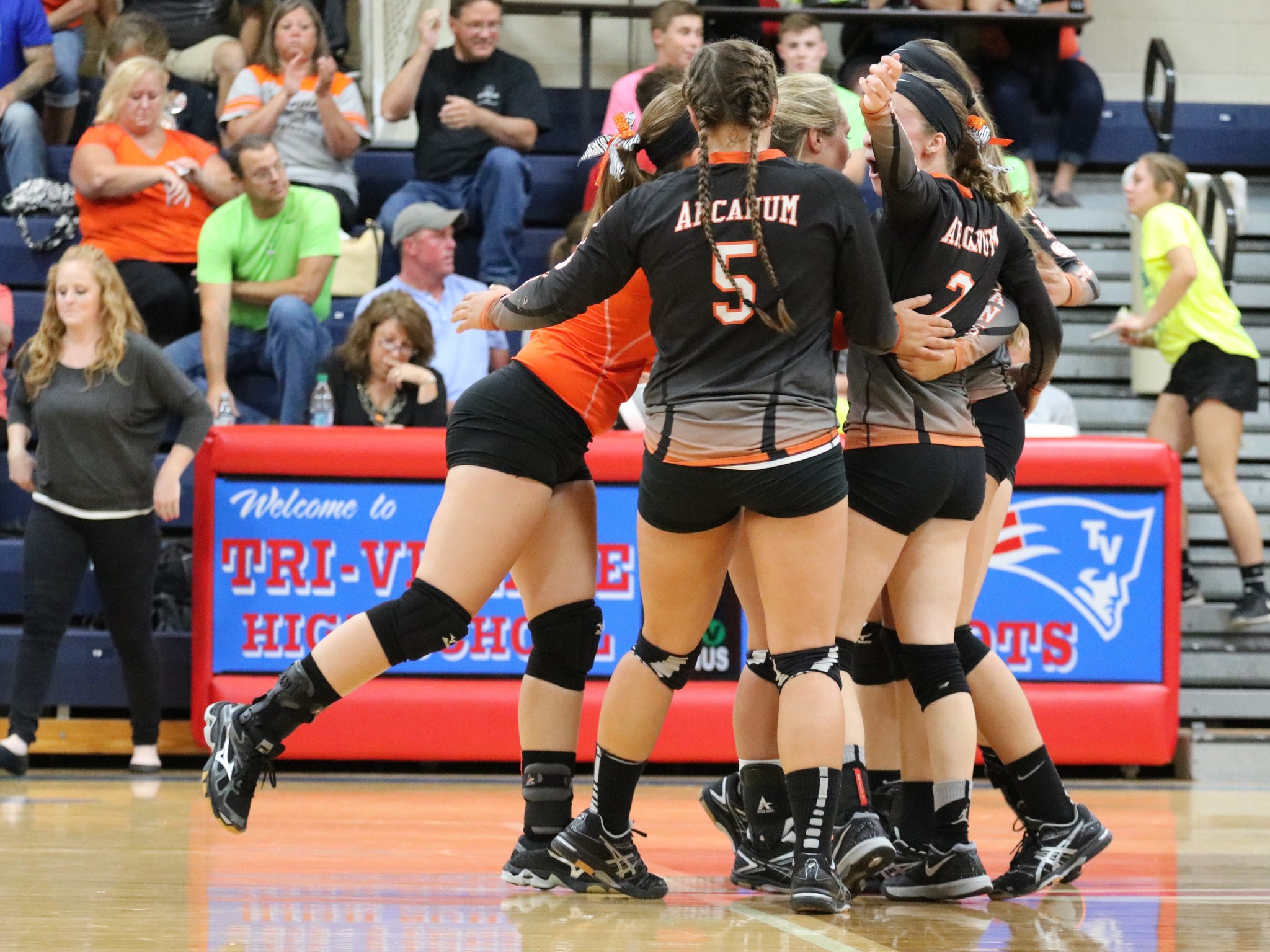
[0,88,609,754]
[1026,98,1270,166]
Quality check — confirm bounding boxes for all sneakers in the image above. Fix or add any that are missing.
[790,852,851,914]
[500,833,620,892]
[201,702,286,835]
[551,808,667,899]
[698,764,993,900]
[988,800,1113,901]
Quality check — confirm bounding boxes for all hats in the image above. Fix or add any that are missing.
[390,200,466,248]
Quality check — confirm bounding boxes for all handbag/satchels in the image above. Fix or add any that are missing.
[2,175,77,254]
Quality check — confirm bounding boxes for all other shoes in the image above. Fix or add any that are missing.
[1047,186,1078,207]
[0,746,27,776]
[1185,570,1203,609]
[130,763,161,773]
[1229,589,1270,625]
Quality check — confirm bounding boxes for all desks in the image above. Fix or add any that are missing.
[191,427,1182,763]
[699,6,1094,154]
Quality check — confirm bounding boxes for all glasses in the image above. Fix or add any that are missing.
[250,161,288,185]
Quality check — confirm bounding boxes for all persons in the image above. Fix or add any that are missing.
[1108,151,1270,624]
[0,0,1112,914]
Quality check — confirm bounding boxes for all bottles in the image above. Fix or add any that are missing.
[308,373,335,427]
[212,391,237,428]
